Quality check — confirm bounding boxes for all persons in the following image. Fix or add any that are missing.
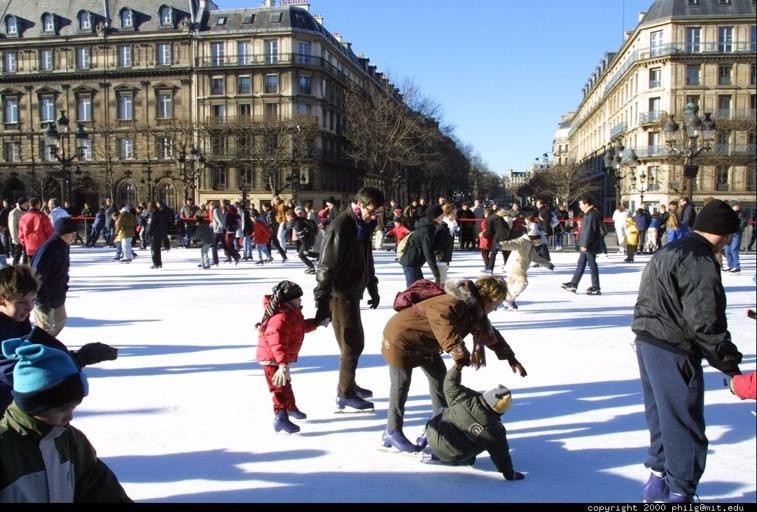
[631,199,743,502]
[0,340,138,504]
[0,183,755,479]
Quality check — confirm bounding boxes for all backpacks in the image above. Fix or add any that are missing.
[394,279,445,319]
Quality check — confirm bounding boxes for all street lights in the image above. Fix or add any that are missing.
[285,169,306,205]
[604,141,637,210]
[49,111,89,204]
[534,153,551,169]
[541,150,562,164]
[664,98,715,205]
[627,169,655,206]
[176,144,205,203]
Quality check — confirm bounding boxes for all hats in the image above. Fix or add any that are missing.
[2,338,84,414]
[277,280,303,302]
[527,223,540,239]
[54,217,78,236]
[18,197,28,205]
[483,385,512,415]
[694,200,741,235]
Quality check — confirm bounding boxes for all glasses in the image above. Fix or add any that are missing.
[366,208,384,216]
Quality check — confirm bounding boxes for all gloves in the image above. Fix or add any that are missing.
[315,303,331,327]
[504,471,524,480]
[456,355,471,368]
[272,365,290,387]
[508,357,527,377]
[76,343,117,366]
[368,293,380,309]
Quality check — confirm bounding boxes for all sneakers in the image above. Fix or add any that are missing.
[563,282,577,289]
[722,267,740,272]
[305,267,315,274]
[150,265,162,268]
[274,409,306,432]
[114,250,137,262]
[643,472,695,503]
[383,428,427,452]
[586,287,600,291]
[198,256,272,268]
[337,386,374,409]
